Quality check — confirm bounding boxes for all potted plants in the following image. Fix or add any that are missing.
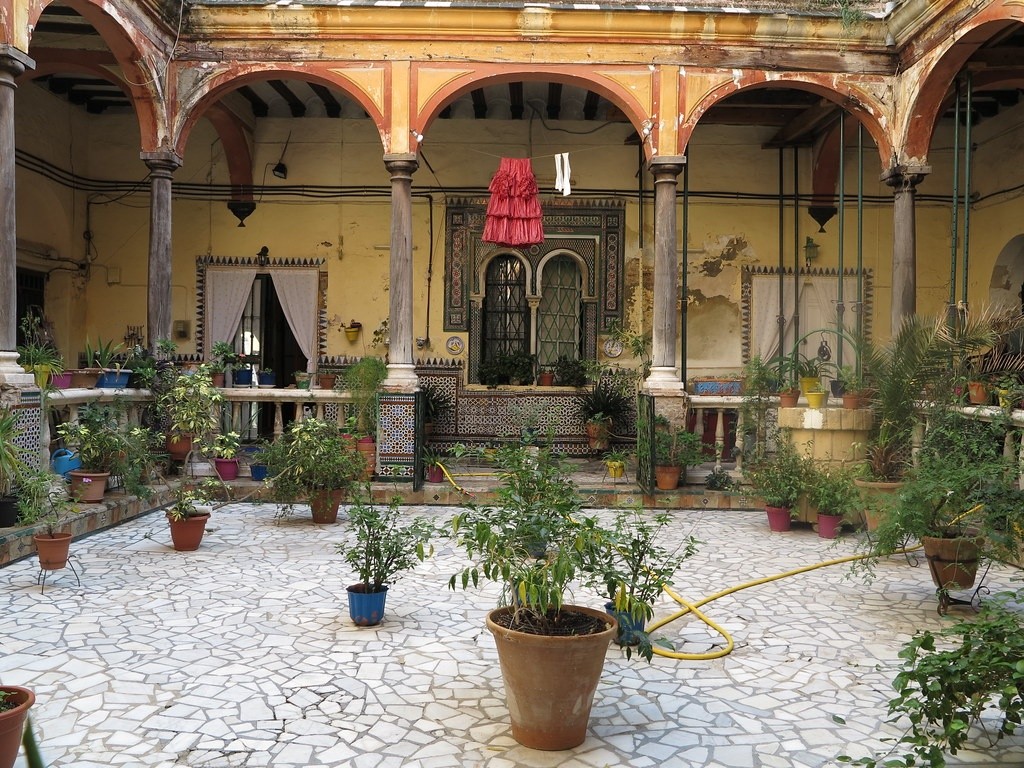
[0,302,262,551]
[294,370,311,389]
[631,414,725,490]
[571,378,637,450]
[332,463,449,626]
[256,368,276,384]
[366,316,390,349]
[344,357,386,435]
[420,420,706,752]
[0,684,35,768]
[475,347,588,388]
[316,368,343,390]
[251,417,363,525]
[689,297,1024,619]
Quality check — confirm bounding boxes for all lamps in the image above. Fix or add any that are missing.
[257,246,269,266]
[803,236,822,258]
[273,162,288,179]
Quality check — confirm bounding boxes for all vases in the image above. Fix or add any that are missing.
[345,328,359,341]
[33,532,72,569]
[230,369,253,385]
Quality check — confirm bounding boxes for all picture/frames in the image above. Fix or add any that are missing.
[342,433,376,482]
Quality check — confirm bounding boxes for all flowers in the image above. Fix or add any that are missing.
[228,354,249,371]
[11,468,92,537]
